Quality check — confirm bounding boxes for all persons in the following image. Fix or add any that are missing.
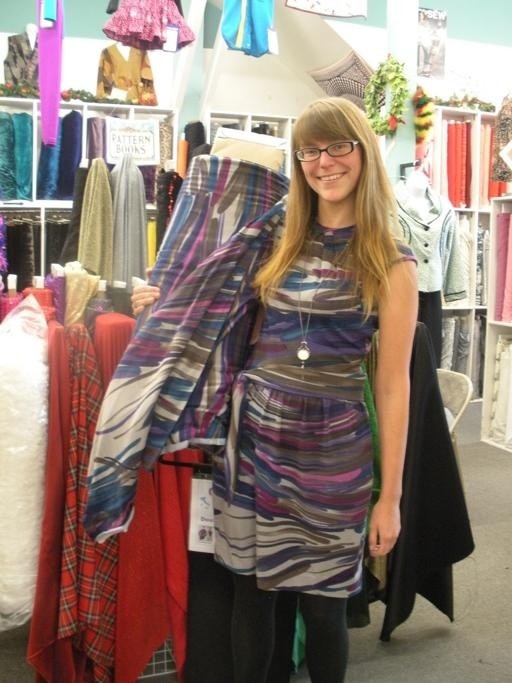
[376,164,468,373]
[209,93,421,682]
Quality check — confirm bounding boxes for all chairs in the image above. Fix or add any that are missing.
[437,367,473,435]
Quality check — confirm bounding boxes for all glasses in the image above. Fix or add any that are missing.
[295,141,358,162]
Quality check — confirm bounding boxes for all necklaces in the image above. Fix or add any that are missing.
[294,219,357,373]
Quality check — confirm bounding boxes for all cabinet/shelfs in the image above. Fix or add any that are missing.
[481,109,512,456]
[201,112,296,159]
[418,94,487,405]
[80,101,180,215]
[1,93,81,309]
